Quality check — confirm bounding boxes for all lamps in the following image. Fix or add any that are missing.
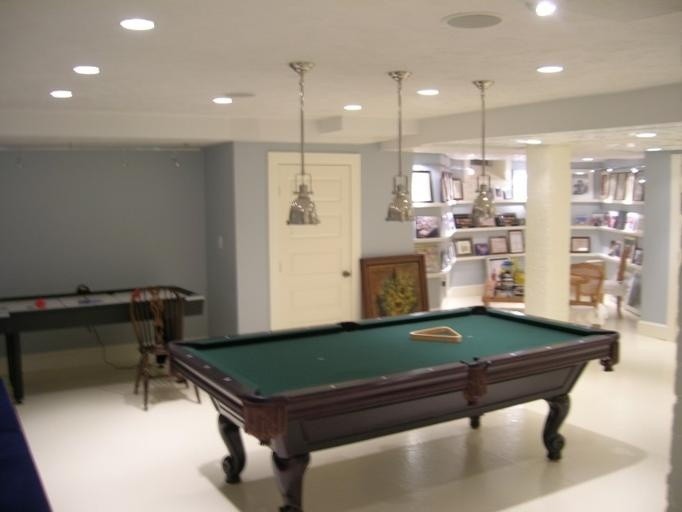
[387,69,423,221]
[282,58,325,229]
[470,81,503,230]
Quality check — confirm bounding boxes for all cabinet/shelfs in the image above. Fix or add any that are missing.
[412,198,645,281]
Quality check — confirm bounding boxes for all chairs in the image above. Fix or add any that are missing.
[129,285,201,411]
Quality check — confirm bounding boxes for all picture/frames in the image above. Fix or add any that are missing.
[361,254,429,320]
[452,177,463,200]
[508,230,524,253]
[570,167,649,305]
[486,257,510,283]
[413,171,433,203]
[453,238,474,257]
[488,236,508,254]
[477,175,490,192]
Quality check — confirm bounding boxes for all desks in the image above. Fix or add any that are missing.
[1,285,205,404]
[168,306,619,511]
